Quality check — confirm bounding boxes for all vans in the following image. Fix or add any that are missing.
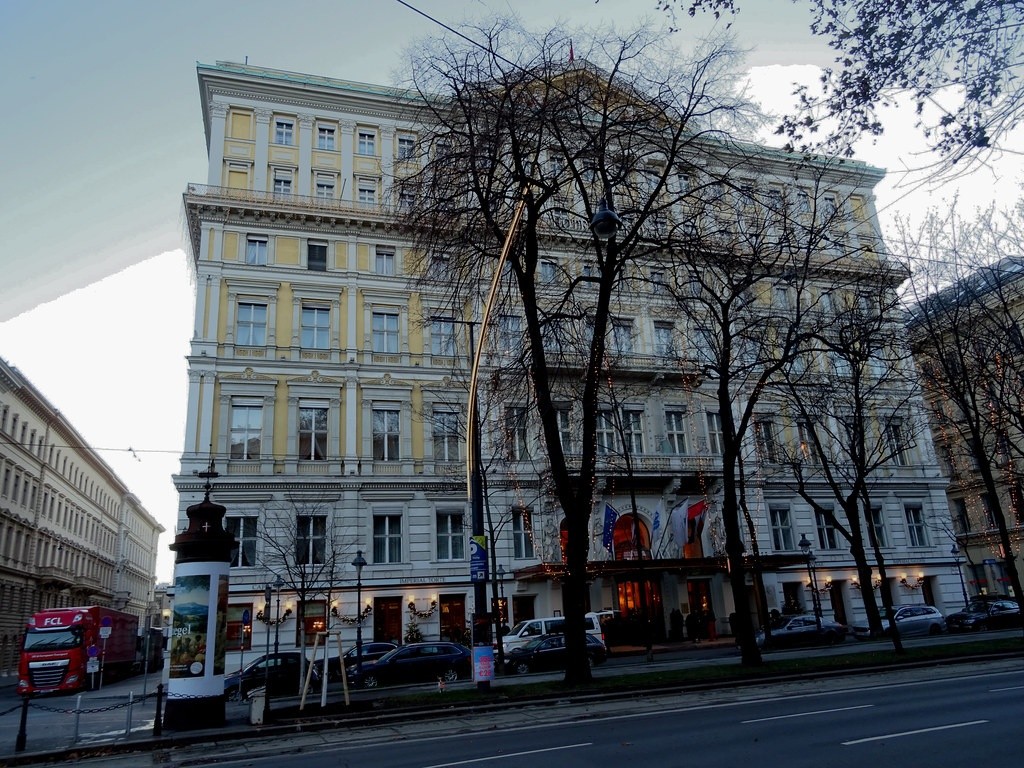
[503,616,605,653]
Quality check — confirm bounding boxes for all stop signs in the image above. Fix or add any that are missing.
[239,608,251,691]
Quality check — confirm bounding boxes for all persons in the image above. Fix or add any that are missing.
[709,517,723,551]
[669,607,718,643]
[601,615,614,653]
[542,518,558,562]
[171,635,206,657]
[502,621,510,635]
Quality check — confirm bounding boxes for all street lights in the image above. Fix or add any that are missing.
[351,550,367,690]
[496,564,506,624]
[273,574,284,696]
[799,532,824,629]
[951,544,969,607]
[809,551,824,617]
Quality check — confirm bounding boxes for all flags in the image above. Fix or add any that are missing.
[671,500,689,547]
[652,499,661,544]
[603,505,617,552]
[688,501,705,519]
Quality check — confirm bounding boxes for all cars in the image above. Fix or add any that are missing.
[756,616,850,652]
[946,601,1020,633]
[224,652,322,693]
[347,642,472,690]
[503,633,608,676]
[852,606,945,641]
[312,642,398,683]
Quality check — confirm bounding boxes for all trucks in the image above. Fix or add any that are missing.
[15,604,140,696]
